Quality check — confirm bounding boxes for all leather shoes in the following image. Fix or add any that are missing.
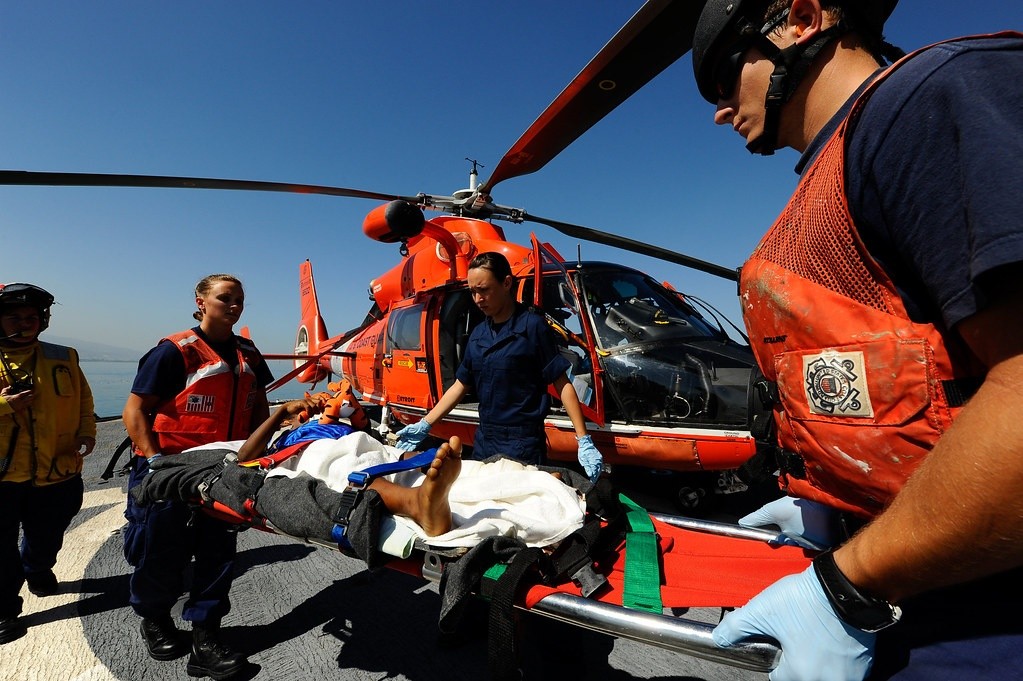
[186,621,250,681]
[140,607,191,661]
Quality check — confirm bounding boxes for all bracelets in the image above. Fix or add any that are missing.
[813,546,903,634]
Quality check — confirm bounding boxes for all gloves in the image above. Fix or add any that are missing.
[574,434,603,483]
[709,561,876,681]
[146,453,163,464]
[394,418,432,452]
[737,495,835,550]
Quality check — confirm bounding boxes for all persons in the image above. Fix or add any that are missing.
[122,274,275,679]
[395,252,602,485]
[237,397,562,537]
[0,282,98,644]
[691,0,1023,681]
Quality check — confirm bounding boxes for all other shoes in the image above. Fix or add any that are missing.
[20,550,58,597]
[0,616,28,645]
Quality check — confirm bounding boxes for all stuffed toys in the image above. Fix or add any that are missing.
[297,379,367,430]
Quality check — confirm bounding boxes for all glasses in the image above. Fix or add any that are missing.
[701,6,792,106]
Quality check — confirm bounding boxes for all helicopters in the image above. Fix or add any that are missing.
[0,0,784,474]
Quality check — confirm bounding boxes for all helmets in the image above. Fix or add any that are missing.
[692,0,898,105]
[0,283,54,331]
